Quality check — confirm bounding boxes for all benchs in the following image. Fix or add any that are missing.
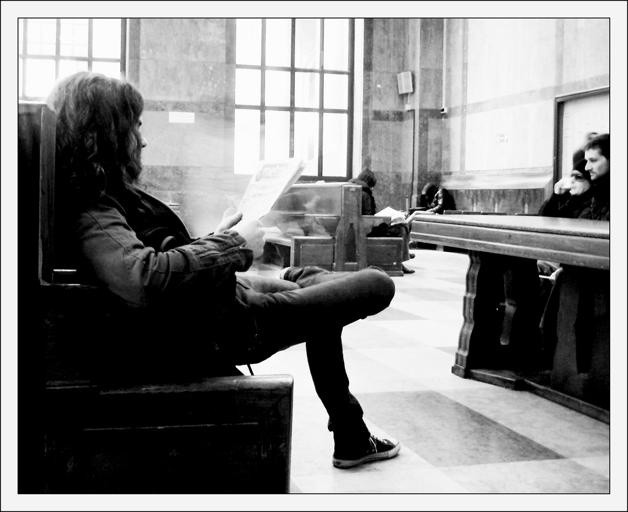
[403,185,550,220]
[260,181,353,271]
[354,183,414,279]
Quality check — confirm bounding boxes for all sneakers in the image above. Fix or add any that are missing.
[333,435,400,468]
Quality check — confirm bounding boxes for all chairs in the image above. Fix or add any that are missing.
[18,95,296,494]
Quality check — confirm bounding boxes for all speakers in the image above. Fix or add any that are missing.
[396,71,414,95]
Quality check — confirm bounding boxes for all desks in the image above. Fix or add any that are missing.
[407,207,609,427]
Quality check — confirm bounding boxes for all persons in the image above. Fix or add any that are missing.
[535,150,593,365]
[420,182,457,215]
[348,165,416,276]
[577,132,609,376]
[52,72,402,471]
